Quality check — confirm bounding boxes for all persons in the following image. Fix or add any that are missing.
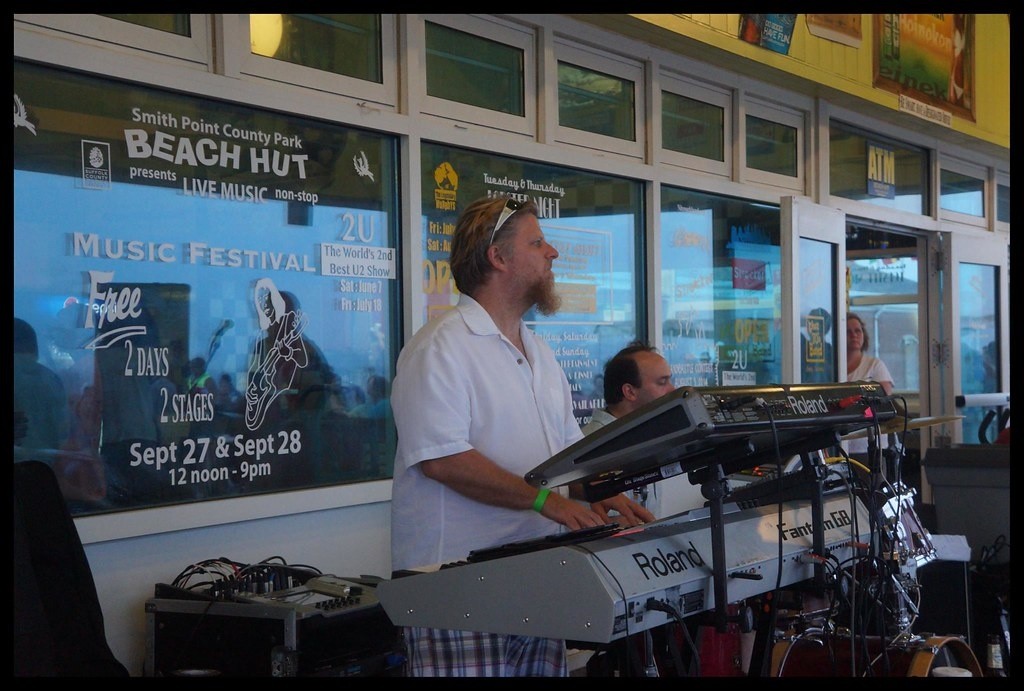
[391,195,655,677]
[578,344,673,678]
[15,291,388,514]
[846,313,904,453]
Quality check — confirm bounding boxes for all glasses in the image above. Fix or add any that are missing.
[490,197,523,246]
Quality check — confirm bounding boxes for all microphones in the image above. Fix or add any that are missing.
[212,319,234,342]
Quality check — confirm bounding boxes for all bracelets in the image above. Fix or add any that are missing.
[533,488,551,511]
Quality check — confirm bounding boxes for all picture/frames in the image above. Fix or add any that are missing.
[872,14,978,125]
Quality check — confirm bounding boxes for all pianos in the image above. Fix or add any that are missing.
[372,378,906,686]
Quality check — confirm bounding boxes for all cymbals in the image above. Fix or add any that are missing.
[840,414,966,443]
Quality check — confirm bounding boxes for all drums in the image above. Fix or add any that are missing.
[743,578,839,624]
[868,481,938,575]
[767,627,985,680]
